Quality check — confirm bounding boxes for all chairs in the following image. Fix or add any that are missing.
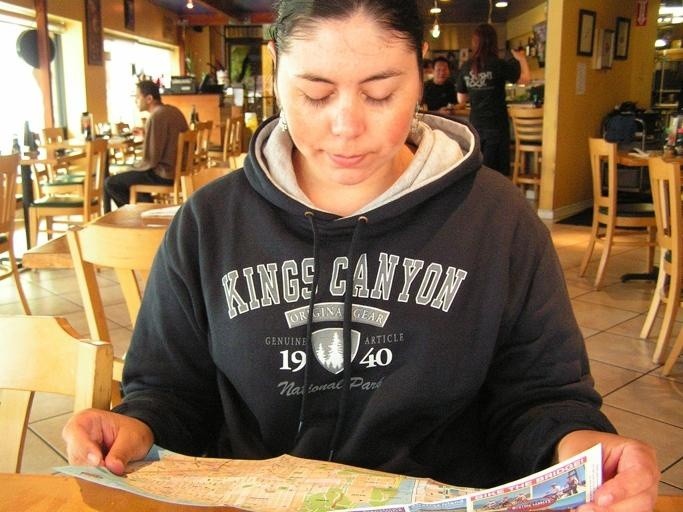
[130,130,199,205]
[43,127,86,196]
[28,140,109,248]
[209,116,242,161]
[0,154,32,317]
[509,108,544,207]
[181,165,234,203]
[0,314,114,473]
[67,224,171,407]
[190,120,214,174]
[578,137,658,292]
[640,158,683,364]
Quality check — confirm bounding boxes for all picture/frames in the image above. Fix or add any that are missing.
[577,9,597,57]
[614,16,631,60]
[598,27,617,69]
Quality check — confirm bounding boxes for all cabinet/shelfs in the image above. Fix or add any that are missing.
[601,110,661,203]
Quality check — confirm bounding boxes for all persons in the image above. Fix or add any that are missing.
[458,23,520,177]
[420,56,458,116]
[104,80,191,207]
[60,0,661,512]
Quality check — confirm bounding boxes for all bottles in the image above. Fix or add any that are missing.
[526,37,531,56]
